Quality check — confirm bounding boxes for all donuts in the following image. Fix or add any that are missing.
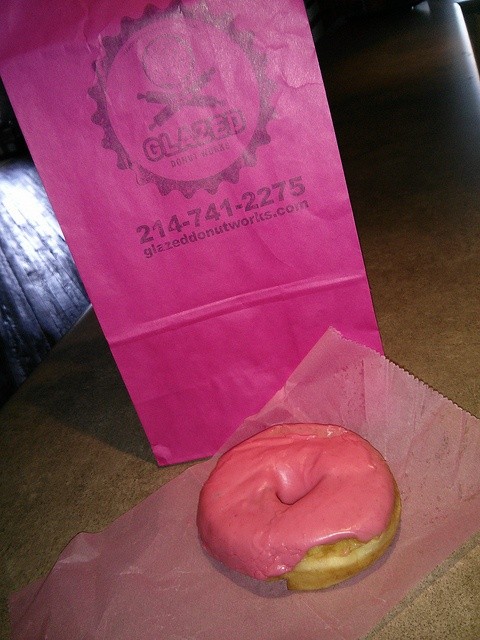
[197,422,400,595]
